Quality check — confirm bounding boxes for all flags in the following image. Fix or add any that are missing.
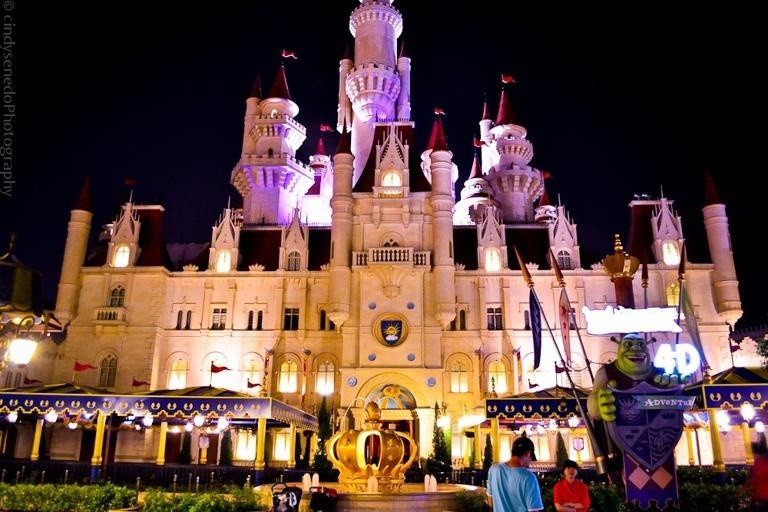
[557,288,576,362]
[527,287,541,371]
[682,283,707,366]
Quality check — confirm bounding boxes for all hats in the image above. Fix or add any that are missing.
[514,437,537,461]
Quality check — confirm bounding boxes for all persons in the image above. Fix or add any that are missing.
[552,459,592,512]
[742,441,768,511]
[486,437,546,512]
